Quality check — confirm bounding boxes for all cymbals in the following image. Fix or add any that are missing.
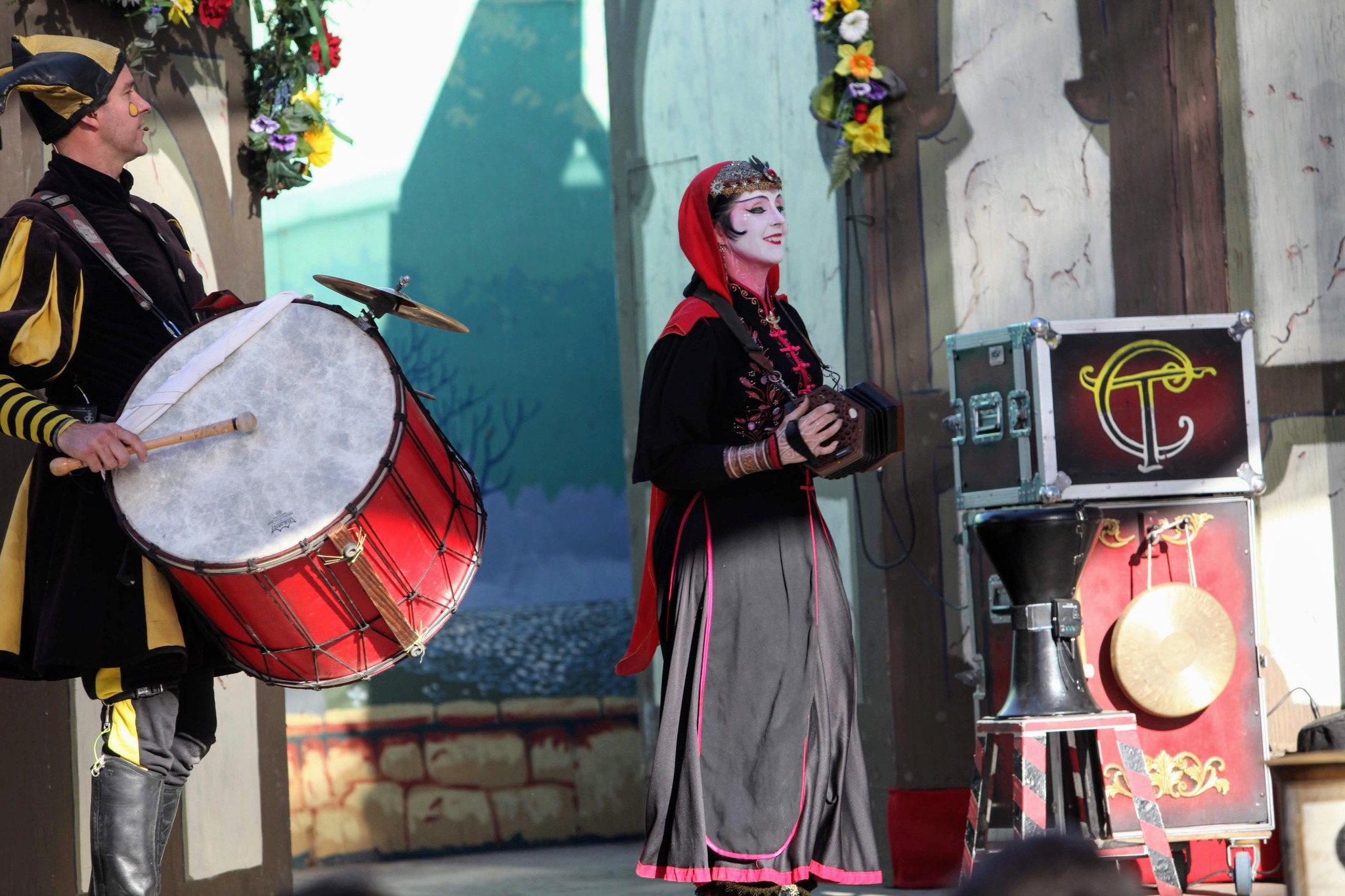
[312,274,470,334]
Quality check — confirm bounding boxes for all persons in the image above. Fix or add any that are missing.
[957,832,1146,896]
[615,156,882,896]
[0,33,245,895]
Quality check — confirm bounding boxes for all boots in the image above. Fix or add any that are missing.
[157,785,183,873]
[89,757,166,896]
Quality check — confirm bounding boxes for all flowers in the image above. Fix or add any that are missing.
[108,0,353,219]
[812,0,896,201]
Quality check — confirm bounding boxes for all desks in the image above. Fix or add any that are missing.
[959,710,1182,895]
[1265,749,1345,896]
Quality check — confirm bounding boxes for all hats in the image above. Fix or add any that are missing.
[710,154,784,197]
[0,34,125,150]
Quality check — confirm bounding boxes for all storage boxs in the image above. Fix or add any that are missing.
[946,311,1265,511]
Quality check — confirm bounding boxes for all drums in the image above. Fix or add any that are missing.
[99,295,487,688]
[972,505,1105,718]
